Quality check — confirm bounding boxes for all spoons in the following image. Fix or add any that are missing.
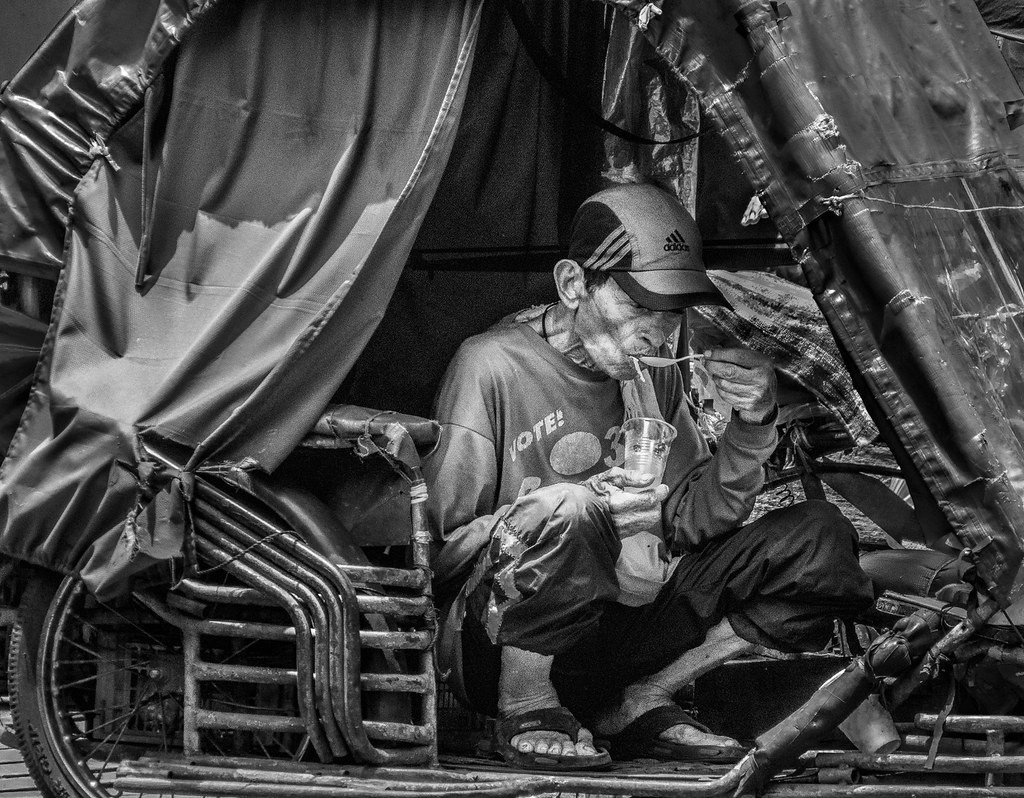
[639,353,706,367]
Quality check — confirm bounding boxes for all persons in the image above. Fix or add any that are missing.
[424,179,875,772]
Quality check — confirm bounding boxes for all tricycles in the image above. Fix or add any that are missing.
[3,390,1024,797]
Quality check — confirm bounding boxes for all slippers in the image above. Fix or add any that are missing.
[493,707,612,770]
[595,705,747,763]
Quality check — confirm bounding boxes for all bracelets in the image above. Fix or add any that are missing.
[760,401,779,425]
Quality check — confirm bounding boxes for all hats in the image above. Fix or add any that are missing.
[567,183,735,312]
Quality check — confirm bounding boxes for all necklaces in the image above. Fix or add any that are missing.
[541,302,560,343]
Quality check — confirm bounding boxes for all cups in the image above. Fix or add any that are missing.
[816,668,901,755]
[621,419,678,495]
